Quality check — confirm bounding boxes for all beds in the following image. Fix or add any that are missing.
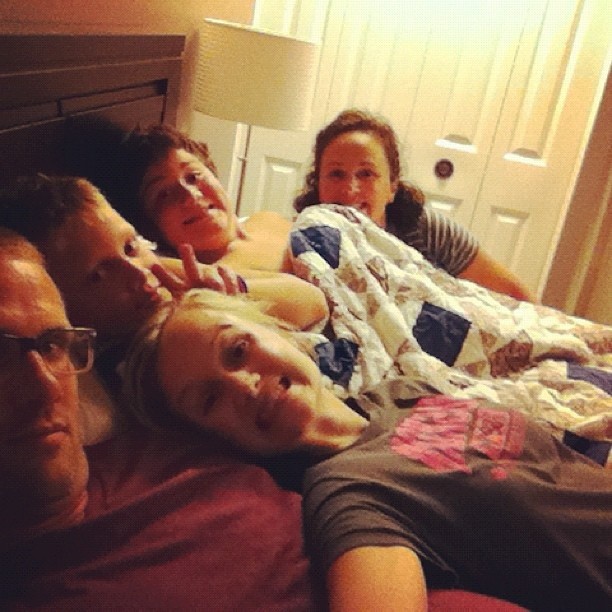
[0,33,187,249]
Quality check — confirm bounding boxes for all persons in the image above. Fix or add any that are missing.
[289,108,543,308]
[102,119,300,278]
[1,172,330,403]
[1,223,533,610]
[115,281,610,610]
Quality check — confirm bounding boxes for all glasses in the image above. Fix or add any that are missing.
[0,327,97,375]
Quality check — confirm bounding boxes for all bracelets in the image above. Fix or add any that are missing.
[230,270,250,303]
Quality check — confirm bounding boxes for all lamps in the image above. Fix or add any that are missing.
[190,17,327,216]
